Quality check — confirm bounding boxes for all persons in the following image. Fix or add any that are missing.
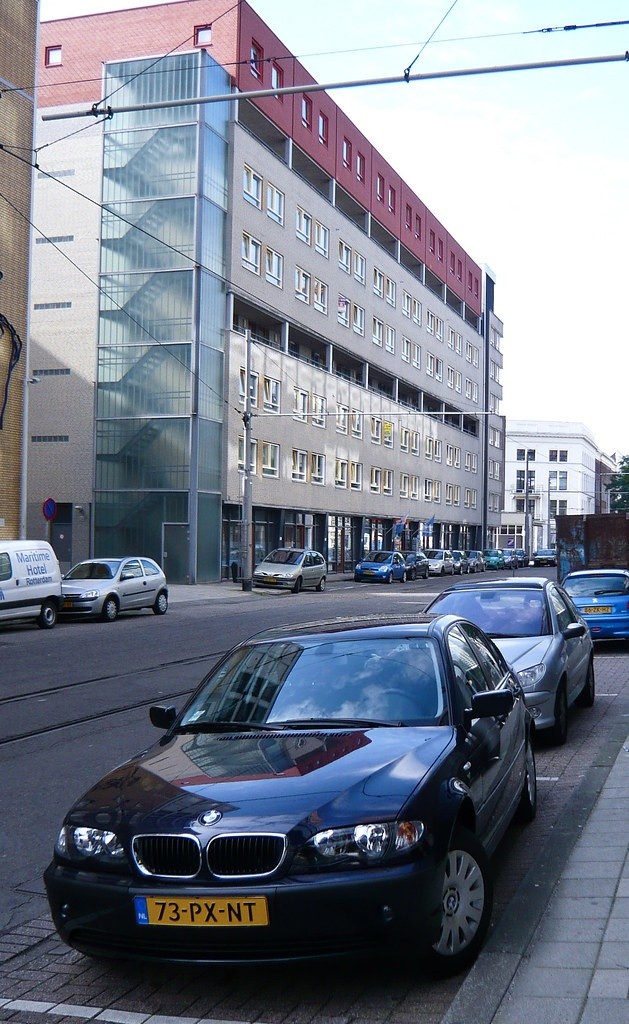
[231,562,238,583]
[253,560,257,577]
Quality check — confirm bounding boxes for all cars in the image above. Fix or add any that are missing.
[552,569,629,644]
[398,551,431,581]
[254,548,328,594]
[498,548,519,570]
[513,548,530,567]
[44,609,536,985]
[482,549,505,570]
[354,551,407,584]
[405,576,596,744]
[54,556,169,622]
[532,548,558,566]
[450,550,470,574]
[465,550,487,572]
[421,549,455,576]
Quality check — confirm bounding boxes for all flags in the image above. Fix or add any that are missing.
[394,513,435,536]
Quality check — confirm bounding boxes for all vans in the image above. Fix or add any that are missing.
[0,540,65,629]
